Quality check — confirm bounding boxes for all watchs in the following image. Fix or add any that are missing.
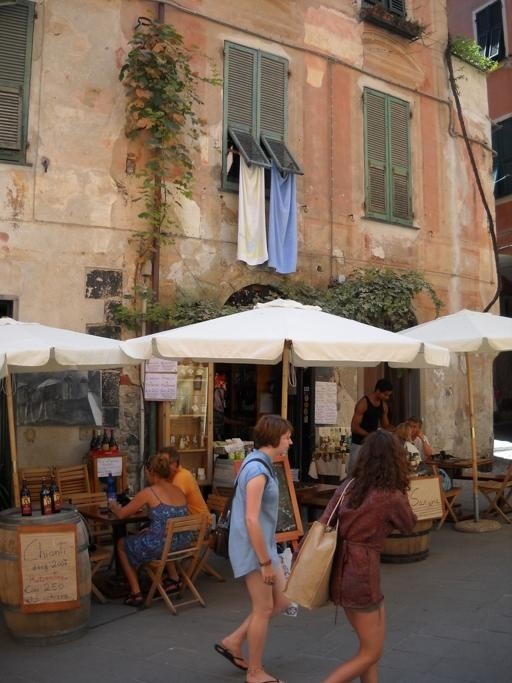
[259,560,272,567]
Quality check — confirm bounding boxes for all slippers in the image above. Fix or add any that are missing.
[244,678,279,683]
[214,643,248,671]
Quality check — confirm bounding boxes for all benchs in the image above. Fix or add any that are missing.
[302,496,330,509]
[452,472,507,482]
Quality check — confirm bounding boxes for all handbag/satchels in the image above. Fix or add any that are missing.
[280,521,338,612]
[438,468,452,491]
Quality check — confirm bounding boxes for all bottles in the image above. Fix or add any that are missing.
[102,429,110,450]
[192,428,199,449]
[109,430,117,449]
[186,432,191,449]
[19,479,32,516]
[49,480,62,513]
[178,432,185,449]
[107,473,118,511]
[40,477,53,514]
[90,429,96,449]
[97,430,103,449]
[170,431,175,447]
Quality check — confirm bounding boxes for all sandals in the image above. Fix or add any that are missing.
[203,527,230,560]
[123,591,144,607]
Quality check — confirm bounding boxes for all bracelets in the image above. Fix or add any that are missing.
[423,438,427,443]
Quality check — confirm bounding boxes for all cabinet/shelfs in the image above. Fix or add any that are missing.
[88,448,128,546]
[239,365,275,426]
[157,360,214,486]
[216,366,239,419]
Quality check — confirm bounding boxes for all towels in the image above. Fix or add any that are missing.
[268,158,298,274]
[236,153,270,266]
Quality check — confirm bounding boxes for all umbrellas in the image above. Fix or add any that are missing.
[117,297,452,420]
[0,316,143,509]
[394,308,512,533]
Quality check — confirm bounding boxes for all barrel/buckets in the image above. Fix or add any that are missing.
[380,475,432,564]
[0,505,92,647]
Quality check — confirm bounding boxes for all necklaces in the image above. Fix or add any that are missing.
[373,399,378,406]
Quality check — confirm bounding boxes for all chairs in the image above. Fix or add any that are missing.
[477,462,512,523]
[17,466,55,502]
[145,514,208,616]
[89,544,111,605]
[189,495,229,584]
[70,492,114,572]
[423,462,463,531]
[53,464,92,502]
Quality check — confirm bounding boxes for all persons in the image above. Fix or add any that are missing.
[214,414,295,683]
[290,426,418,683]
[347,378,397,477]
[107,455,194,607]
[157,447,213,589]
[404,415,432,462]
[395,423,452,491]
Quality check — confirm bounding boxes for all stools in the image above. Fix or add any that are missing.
[128,526,141,536]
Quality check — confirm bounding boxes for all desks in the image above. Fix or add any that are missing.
[437,457,494,467]
[77,501,150,600]
[293,480,339,509]
